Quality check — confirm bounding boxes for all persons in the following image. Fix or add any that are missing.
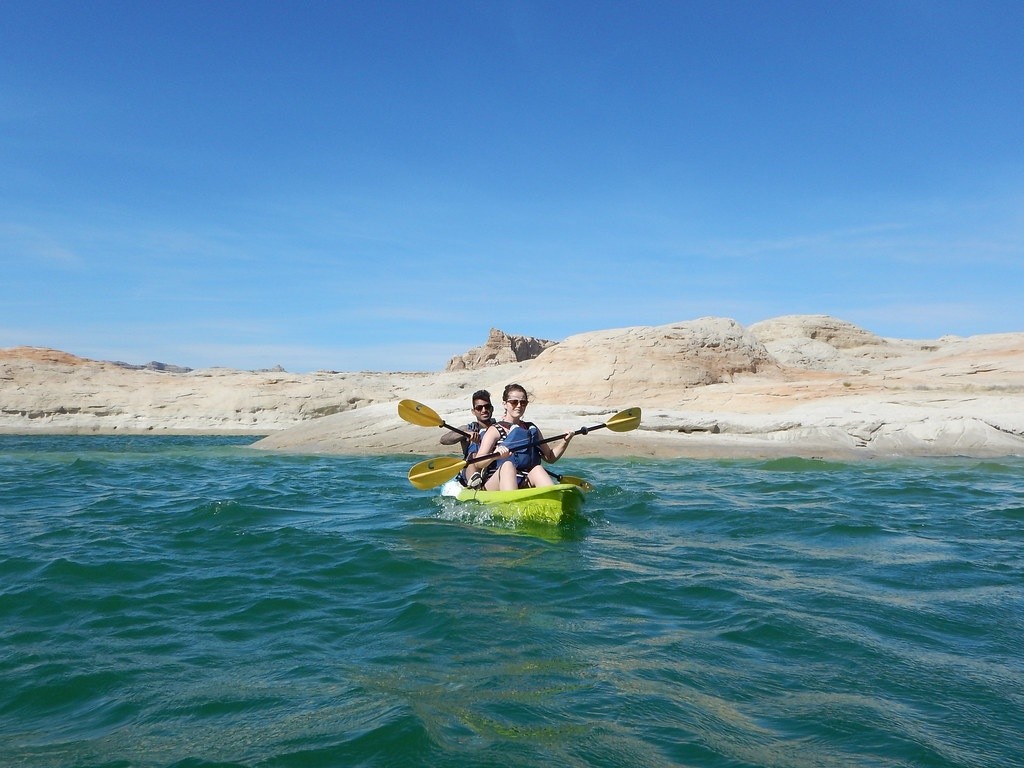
[475,384,575,491]
[440,390,506,488]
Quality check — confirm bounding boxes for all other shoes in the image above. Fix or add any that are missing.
[468,472,483,490]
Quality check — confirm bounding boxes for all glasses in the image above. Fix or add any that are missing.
[506,400,530,406]
[475,404,492,411]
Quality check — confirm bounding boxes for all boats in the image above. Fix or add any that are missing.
[441,478,584,526]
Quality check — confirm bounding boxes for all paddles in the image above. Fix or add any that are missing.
[396,398,593,489]
[408,407,641,490]
